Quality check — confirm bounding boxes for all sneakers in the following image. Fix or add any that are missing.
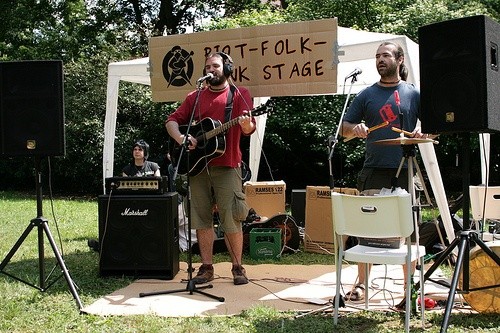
[192,263,215,284]
[231,264,248,285]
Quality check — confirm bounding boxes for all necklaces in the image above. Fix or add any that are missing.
[210,84,228,91]
[380,79,401,84]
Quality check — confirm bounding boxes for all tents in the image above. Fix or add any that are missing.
[103,26,458,263]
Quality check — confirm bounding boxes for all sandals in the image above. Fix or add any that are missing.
[344,282,366,301]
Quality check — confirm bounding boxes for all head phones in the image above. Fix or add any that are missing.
[203,50,236,76]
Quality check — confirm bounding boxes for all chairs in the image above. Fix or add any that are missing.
[331,193,425,333]
[469,185,500,245]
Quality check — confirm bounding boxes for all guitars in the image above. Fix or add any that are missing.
[169,100,278,178]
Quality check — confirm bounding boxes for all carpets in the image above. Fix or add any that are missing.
[80,262,480,317]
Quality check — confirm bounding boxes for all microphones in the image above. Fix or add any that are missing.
[197,73,213,82]
[346,67,363,80]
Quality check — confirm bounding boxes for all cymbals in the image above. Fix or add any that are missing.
[373,138,438,145]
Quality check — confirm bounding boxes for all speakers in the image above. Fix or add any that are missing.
[418,14,500,134]
[0,60,65,155]
[97,192,179,280]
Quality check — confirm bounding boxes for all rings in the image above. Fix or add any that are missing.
[242,122,244,123]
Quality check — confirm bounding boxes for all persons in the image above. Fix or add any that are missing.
[166,52,256,285]
[338,42,440,302]
[122,139,160,176]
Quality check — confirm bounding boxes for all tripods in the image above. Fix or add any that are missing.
[394,136,500,333]
[0,155,83,312]
[138,83,225,302]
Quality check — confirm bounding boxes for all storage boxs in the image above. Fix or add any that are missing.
[305,185,357,255]
[249,228,282,260]
[244,179,286,223]
